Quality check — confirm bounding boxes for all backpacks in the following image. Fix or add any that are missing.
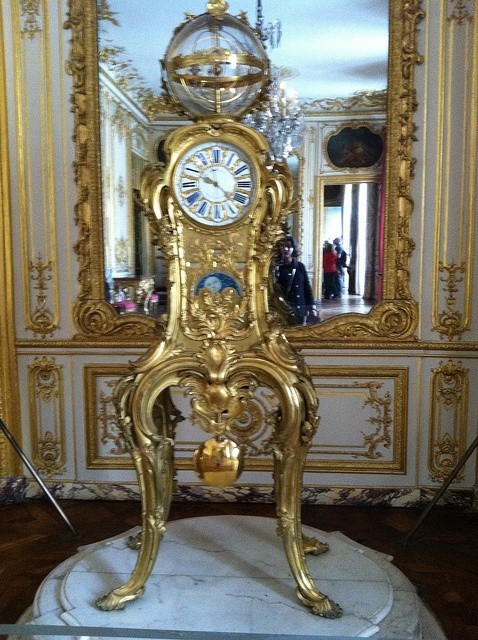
[339,250,350,268]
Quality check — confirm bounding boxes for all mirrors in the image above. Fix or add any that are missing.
[62,0,424,344]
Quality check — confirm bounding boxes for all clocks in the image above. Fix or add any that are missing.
[96,0,342,618]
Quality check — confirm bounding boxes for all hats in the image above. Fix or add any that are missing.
[332,238,341,245]
[322,241,328,246]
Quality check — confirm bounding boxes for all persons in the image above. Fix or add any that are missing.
[203,277,223,293]
[333,238,347,295]
[275,236,317,327]
[323,243,341,303]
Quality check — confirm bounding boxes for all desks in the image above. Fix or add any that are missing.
[8,514,446,640]
